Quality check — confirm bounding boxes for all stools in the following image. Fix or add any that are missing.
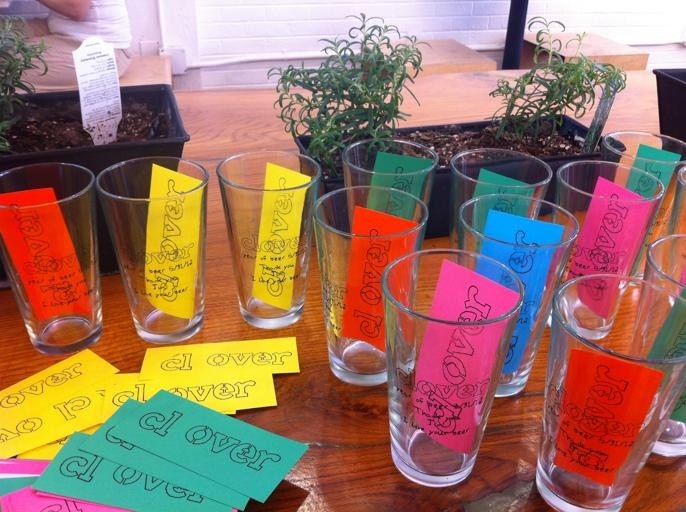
[364,36,496,78]
[522,31,649,72]
[120,54,173,90]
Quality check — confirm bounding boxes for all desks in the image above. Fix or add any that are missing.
[0,69,686,512]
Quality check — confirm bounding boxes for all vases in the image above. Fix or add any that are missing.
[651,68,686,162]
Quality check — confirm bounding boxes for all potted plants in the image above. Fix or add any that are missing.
[263,11,626,240]
[0,16,189,275]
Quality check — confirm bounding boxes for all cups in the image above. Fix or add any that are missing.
[631,236,685,458]
[457,194,581,400]
[0,131,686,512]
[536,274,686,510]
[96,158,209,343]
[0,162,95,353]
[380,249,525,489]
[556,161,666,342]
[216,151,321,331]
[311,185,428,382]
[446,148,556,271]
[342,140,441,294]
[598,130,686,287]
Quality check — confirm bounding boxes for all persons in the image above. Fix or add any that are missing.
[0,0,133,92]
